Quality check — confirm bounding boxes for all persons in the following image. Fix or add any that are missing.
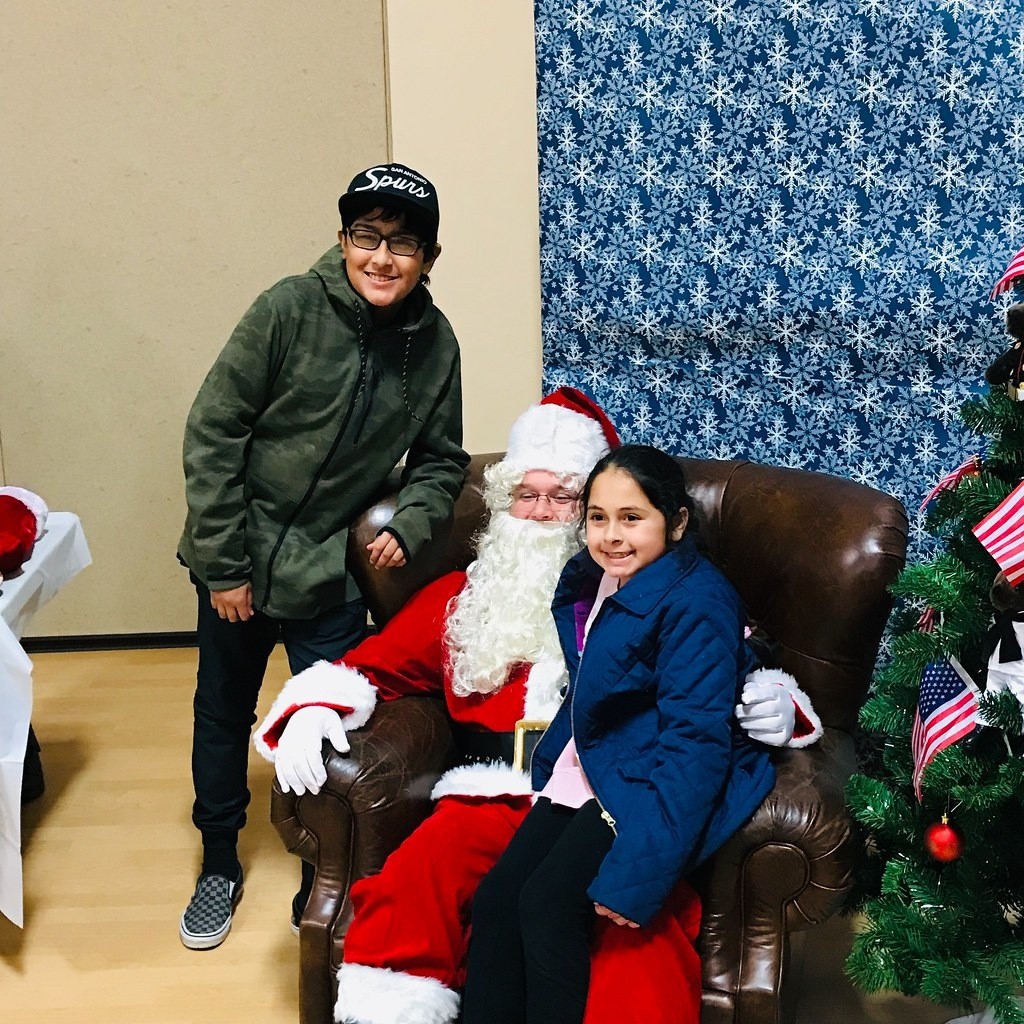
[176,161,472,953]
[452,442,779,1023]
[252,384,825,1024]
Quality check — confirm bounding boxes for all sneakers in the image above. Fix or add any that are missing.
[181,861,244,949]
[290,892,305,937]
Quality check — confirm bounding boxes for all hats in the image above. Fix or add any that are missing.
[338,164,440,234]
[505,386,622,477]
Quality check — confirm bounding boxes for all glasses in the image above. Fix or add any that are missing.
[513,491,581,511]
[346,226,428,256]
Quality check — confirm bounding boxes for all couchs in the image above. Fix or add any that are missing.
[269,453,911,1024]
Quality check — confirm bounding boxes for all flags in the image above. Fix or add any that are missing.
[919,457,976,510]
[912,648,988,804]
[972,478,1024,587]
[987,245,1024,302]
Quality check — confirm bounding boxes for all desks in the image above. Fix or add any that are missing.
[0,512,91,929]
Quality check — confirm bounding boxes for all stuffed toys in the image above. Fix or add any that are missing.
[987,305,1024,479]
[981,568,1024,705]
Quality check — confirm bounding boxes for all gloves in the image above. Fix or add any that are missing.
[273,707,350,796]
[735,682,796,747]
